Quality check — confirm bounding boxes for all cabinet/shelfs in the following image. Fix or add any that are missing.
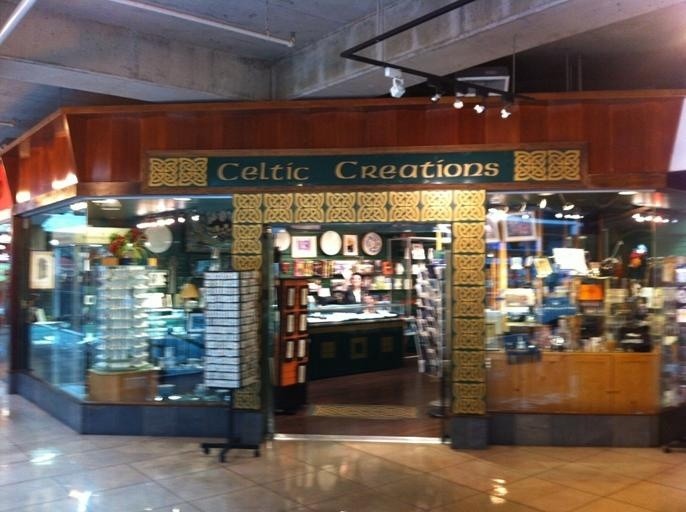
[386,234,450,384]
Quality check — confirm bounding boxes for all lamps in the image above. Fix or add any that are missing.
[385,65,407,98]
[424,74,516,120]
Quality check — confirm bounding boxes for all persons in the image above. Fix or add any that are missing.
[344,272,367,304]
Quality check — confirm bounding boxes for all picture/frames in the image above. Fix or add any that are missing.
[28,247,57,293]
